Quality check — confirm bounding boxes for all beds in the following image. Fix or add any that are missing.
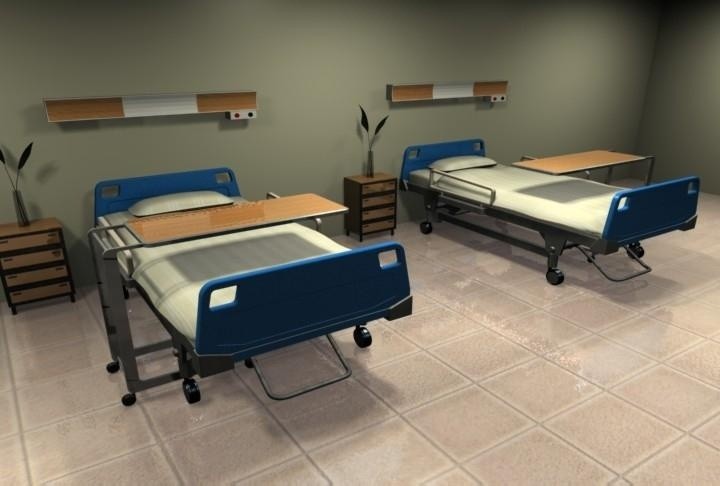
[397,136,701,285]
[90,165,414,403]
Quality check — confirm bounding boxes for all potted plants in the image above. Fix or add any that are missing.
[0,139,35,227]
[354,104,390,177]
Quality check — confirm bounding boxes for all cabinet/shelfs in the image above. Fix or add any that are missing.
[0,218,76,315]
[344,171,398,242]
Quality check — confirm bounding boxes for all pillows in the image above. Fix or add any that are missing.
[424,155,499,171]
[126,189,233,219]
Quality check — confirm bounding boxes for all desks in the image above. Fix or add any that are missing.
[87,189,353,407]
[511,147,655,185]
